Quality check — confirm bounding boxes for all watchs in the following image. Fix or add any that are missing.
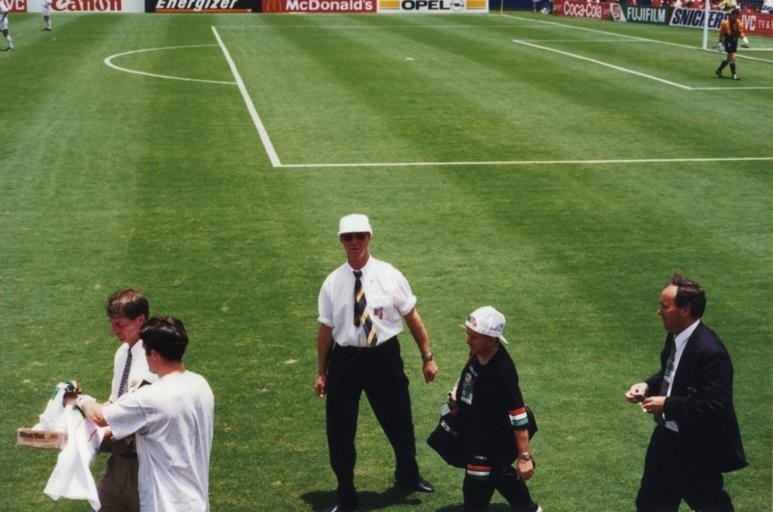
[517,450,532,460]
[421,352,434,361]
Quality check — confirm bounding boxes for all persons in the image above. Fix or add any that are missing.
[426,304,544,512]
[626,0,773,14]
[89,286,161,511]
[714,7,751,81]
[63,315,216,511]
[624,272,748,511]
[0,0,15,52]
[313,213,439,511]
[41,0,53,31]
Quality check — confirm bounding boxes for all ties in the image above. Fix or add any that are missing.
[116,348,132,399]
[659,341,676,397]
[353,271,378,346]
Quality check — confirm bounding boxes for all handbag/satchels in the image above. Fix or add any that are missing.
[427,405,538,468]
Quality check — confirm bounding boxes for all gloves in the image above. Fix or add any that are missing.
[717,42,723,52]
[740,37,751,50]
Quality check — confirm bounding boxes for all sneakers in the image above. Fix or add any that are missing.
[714,69,740,81]
[42,27,52,32]
[5,47,14,52]
[535,505,543,512]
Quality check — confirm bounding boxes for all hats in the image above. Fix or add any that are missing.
[465,303,509,344]
[338,214,374,237]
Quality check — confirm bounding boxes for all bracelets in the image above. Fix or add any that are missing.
[78,394,98,410]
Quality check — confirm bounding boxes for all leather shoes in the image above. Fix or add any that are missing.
[326,492,358,512]
[397,473,435,493]
